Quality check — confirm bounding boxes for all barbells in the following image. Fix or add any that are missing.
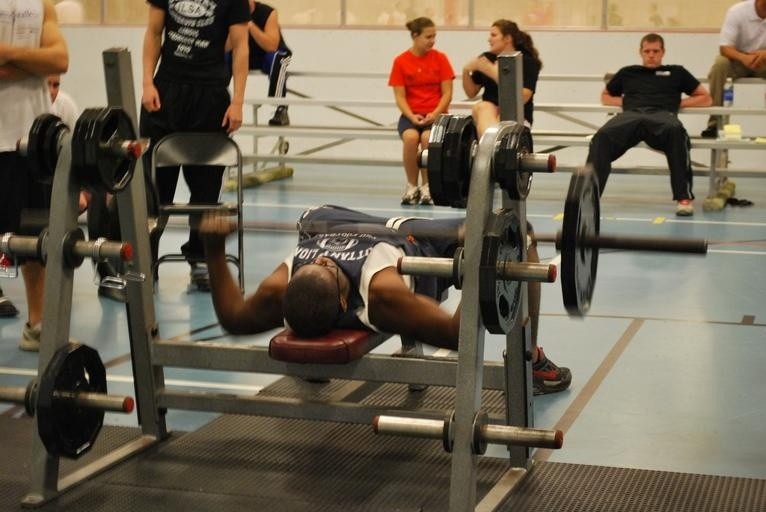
[99,165,706,318]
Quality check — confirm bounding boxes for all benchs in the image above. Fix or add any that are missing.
[225,68,766,213]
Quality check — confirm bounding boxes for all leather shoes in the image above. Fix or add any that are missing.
[701,126,717,138]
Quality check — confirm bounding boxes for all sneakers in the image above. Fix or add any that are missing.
[675,198,693,217]
[18,323,76,351]
[532,347,572,396]
[400,182,434,206]
[189,262,211,292]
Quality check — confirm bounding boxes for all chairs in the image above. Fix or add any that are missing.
[149,132,245,298]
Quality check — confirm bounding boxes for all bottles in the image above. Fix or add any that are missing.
[723,77,734,107]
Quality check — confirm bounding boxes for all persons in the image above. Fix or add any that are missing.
[224,0,292,127]
[389,17,457,206]
[589,34,717,217]
[462,20,543,142]
[46,73,79,130]
[0,0,80,355]
[137,0,251,294]
[200,204,573,395]
[702,1,766,137]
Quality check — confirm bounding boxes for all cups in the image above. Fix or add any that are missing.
[718,123,742,140]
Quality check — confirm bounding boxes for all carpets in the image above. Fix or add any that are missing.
[0,352,766,512]
